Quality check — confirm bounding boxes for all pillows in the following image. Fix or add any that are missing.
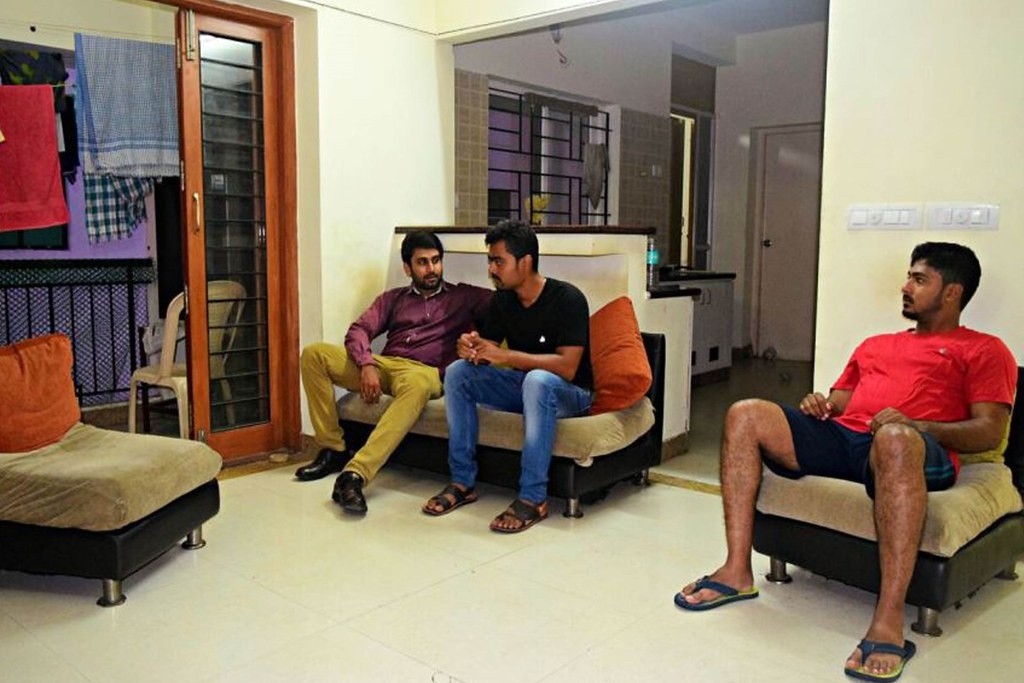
[0,333,84,454]
[586,297,654,417]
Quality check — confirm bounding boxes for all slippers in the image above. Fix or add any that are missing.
[844,640,916,683]
[674,576,759,609]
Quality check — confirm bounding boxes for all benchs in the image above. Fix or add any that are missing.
[337,331,667,519]
[0,421,222,608]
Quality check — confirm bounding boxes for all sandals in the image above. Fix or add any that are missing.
[423,484,478,515]
[490,498,553,532]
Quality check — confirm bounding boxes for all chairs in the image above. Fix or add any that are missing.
[128,280,248,437]
[752,366,1024,638]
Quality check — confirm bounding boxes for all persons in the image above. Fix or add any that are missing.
[675,242,1018,683]
[422,220,594,533]
[295,230,497,514]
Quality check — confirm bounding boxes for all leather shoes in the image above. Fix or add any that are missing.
[295,447,349,479]
[331,471,368,512]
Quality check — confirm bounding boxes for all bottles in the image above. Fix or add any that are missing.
[645,226,659,287]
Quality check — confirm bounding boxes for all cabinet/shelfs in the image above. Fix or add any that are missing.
[690,278,736,377]
